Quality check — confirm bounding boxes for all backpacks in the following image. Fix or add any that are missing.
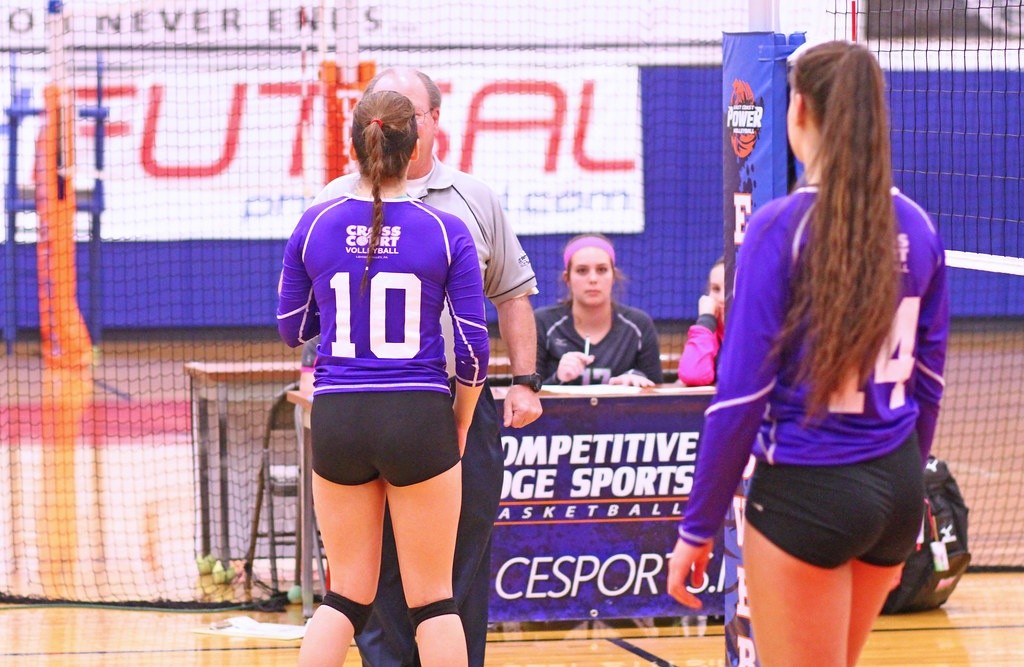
[878,454,969,615]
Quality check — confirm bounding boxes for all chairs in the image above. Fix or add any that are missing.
[247,383,308,600]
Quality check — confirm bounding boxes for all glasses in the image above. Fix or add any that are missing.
[414,107,433,124]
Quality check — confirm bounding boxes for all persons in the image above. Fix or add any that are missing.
[275,92,491,667]
[299,336,321,393]
[678,258,727,390]
[667,42,950,667]
[310,68,543,667]
[533,234,663,388]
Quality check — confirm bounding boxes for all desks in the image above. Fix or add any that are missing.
[278,383,330,615]
[456,384,742,632]
[478,346,520,387]
[651,343,694,374]
[175,348,318,603]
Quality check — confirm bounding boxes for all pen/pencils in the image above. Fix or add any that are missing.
[585,336,590,355]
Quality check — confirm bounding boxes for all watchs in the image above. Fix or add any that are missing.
[512,373,544,393]
